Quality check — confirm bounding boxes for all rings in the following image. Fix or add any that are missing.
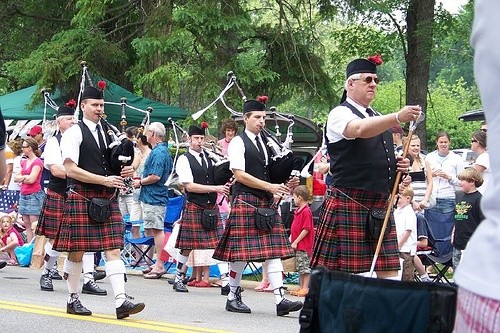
[412,115,416,118]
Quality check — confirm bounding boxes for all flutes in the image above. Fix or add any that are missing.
[99,114,134,176]
[200,145,234,185]
[261,127,304,184]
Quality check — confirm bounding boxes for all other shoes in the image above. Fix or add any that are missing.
[414,273,430,283]
[0,261,7,269]
[142,263,309,297]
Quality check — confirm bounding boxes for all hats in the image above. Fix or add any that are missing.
[188,125,205,137]
[27,125,42,136]
[243,100,266,114]
[56,106,75,118]
[346,58,377,79]
[81,86,104,100]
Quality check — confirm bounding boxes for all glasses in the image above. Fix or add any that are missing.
[353,76,379,84]
[472,140,477,143]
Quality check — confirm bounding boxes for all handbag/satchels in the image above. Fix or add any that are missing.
[89,198,112,223]
[202,209,218,229]
[366,207,391,240]
[14,236,36,266]
[255,208,276,230]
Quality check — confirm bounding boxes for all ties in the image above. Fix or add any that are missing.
[199,153,207,168]
[95,123,107,153]
[367,107,375,116]
[255,135,266,163]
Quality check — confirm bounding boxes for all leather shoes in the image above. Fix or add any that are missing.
[51,271,62,280]
[276,298,303,316]
[40,274,53,291]
[81,280,107,296]
[66,299,92,315]
[116,300,145,319]
[93,270,106,280]
[226,298,251,313]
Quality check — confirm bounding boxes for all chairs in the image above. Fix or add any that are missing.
[125,194,185,270]
[420,209,458,284]
[300,266,458,333]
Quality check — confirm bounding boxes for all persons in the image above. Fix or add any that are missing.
[256,184,314,296]
[452,0,500,333]
[168,118,243,296]
[60,85,145,320]
[319,127,489,284]
[212,100,302,316]
[0,106,108,296]
[308,59,422,279]
[119,121,173,278]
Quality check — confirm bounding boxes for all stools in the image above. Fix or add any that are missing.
[99,222,133,263]
[242,260,261,282]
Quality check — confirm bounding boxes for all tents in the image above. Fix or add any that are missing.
[0,76,187,127]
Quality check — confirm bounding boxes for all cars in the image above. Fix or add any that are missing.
[218,109,324,170]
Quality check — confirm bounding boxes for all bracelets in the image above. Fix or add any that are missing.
[396,113,400,124]
[140,181,142,185]
